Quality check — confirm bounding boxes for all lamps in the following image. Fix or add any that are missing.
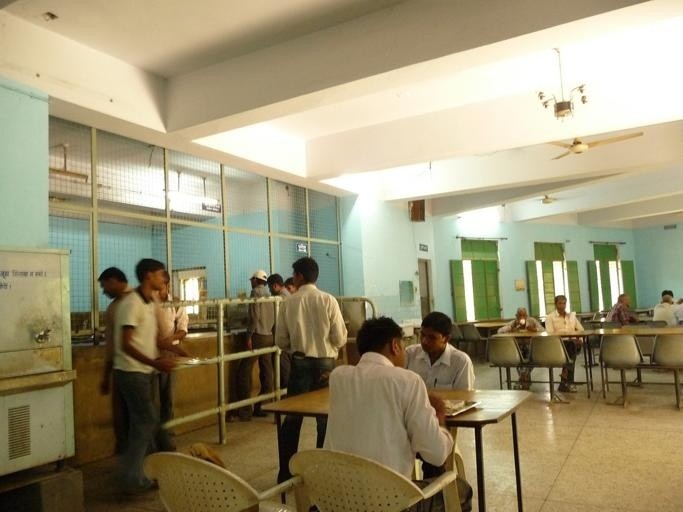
[531,39,592,120]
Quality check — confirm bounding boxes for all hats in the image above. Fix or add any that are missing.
[249,269,268,283]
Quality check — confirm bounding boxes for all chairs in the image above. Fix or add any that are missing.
[435,308,682,411]
[139,447,303,512]
[284,449,462,512]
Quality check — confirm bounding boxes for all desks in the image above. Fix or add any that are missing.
[259,383,536,512]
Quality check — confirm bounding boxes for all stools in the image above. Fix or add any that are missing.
[414,445,468,482]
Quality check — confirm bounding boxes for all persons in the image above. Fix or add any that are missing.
[605,293,638,326]
[405,312,476,480]
[277,259,349,508]
[153,267,186,453]
[284,276,296,293]
[267,271,291,300]
[497,307,544,391]
[651,289,683,327]
[545,295,587,393]
[98,268,142,455]
[321,314,473,512]
[114,257,189,499]
[228,270,275,419]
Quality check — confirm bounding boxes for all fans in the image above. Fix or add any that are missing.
[526,192,584,204]
[540,130,647,161]
